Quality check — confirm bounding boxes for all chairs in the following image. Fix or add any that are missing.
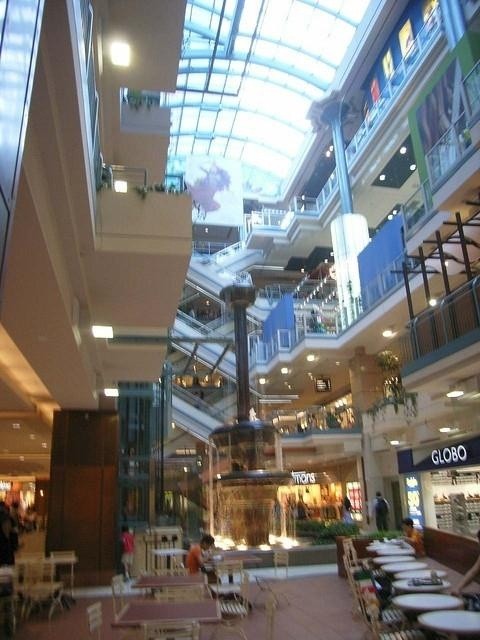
[0,522,291,638]
[341,538,480,639]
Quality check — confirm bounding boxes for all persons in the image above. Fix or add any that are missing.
[275,502,310,520]
[1,500,38,595]
[308,310,320,333]
[369,491,390,531]
[343,506,353,527]
[185,534,226,599]
[120,524,134,580]
[452,531,480,595]
[345,495,351,511]
[398,518,425,558]
[193,378,203,409]
[297,412,317,433]
